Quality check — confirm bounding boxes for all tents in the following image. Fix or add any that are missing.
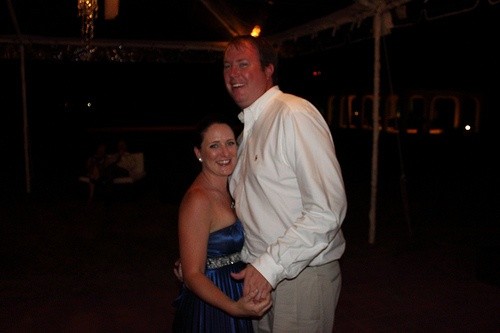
[0,0,500,245]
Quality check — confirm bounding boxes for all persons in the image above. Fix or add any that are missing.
[169,116,273,333]
[74,137,141,216]
[175,33,349,333]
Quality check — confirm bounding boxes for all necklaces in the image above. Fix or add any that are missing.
[199,172,236,210]
